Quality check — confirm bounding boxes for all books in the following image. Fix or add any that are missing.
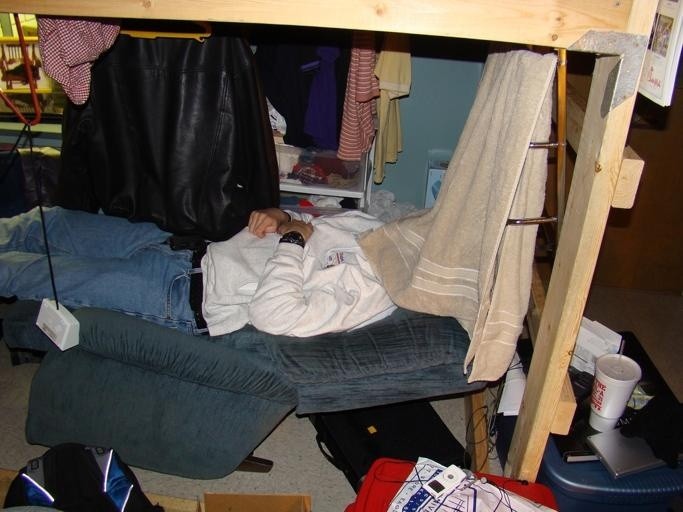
[553,401,602,463]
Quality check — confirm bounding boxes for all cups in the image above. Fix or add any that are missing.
[589,353,642,433]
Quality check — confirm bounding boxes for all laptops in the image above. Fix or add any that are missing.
[586,427,683,481]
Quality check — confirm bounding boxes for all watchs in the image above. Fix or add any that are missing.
[279,230,305,248]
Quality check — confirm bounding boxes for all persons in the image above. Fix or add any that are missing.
[0,205,399,339]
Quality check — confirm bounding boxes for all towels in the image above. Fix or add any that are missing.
[37,16,120,107]
[372,52,412,185]
[337,52,381,164]
[352,52,567,383]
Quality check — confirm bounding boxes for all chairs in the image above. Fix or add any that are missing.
[2,217,516,481]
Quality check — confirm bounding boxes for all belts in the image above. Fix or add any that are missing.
[190,240,210,338]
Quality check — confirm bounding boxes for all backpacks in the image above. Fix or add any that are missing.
[2,442,166,512]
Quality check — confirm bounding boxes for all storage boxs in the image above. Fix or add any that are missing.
[145,495,317,512]
